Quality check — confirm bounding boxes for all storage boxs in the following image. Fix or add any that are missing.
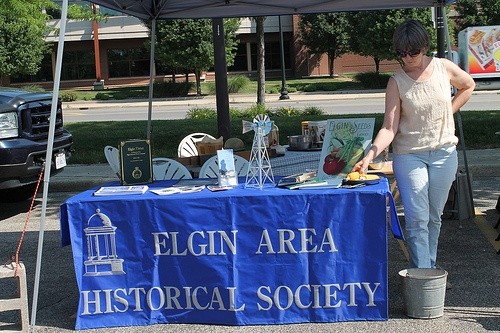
[459,25,500,75]
[302,121,327,142]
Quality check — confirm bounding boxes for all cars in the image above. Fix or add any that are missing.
[0,89,75,203]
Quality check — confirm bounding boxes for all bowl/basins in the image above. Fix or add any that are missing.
[286,135,314,151]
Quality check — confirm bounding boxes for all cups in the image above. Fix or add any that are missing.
[316,141,324,148]
[261,137,269,147]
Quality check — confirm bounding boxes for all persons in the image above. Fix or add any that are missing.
[352,20,476,268]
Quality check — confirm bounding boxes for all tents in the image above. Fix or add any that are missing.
[30,0,476,333]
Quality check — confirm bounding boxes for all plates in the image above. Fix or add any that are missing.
[343,177,382,184]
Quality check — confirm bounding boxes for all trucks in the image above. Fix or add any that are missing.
[430,25,500,97]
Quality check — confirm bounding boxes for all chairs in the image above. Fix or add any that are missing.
[178,132,217,158]
[370,160,411,262]
[199,155,258,178]
[151,158,193,180]
[104,145,121,182]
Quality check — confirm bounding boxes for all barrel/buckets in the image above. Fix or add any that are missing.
[398,267,449,319]
[195,141,222,166]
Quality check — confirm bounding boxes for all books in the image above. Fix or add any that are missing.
[277,173,342,189]
[94,185,148,195]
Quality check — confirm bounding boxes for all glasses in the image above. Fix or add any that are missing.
[396,48,421,58]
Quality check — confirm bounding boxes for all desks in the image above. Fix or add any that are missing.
[60,176,405,330]
[186,144,321,175]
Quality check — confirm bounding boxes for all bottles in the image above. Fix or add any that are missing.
[269,121,280,149]
